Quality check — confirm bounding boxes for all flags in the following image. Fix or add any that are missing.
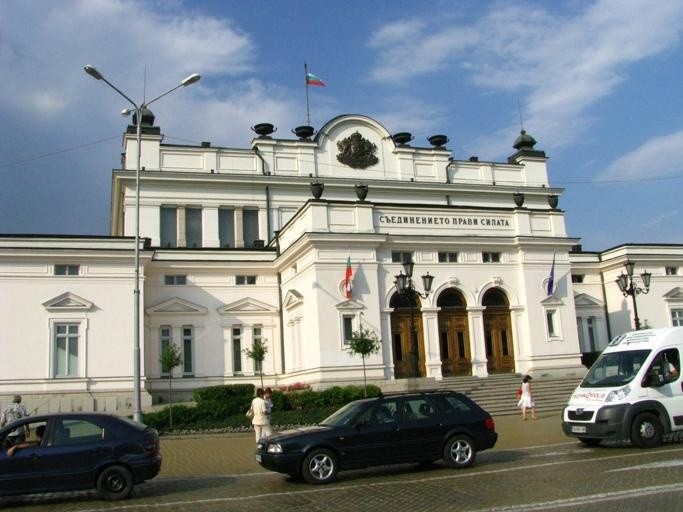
[547,254,556,296]
[345,255,354,298]
[305,73,326,87]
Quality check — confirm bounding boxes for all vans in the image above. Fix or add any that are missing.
[561,325,683,449]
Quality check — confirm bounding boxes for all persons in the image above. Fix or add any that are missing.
[369,406,396,423]
[5,423,44,456]
[652,360,677,382]
[0,395,29,450]
[259,387,273,439]
[250,388,272,444]
[516,374,538,420]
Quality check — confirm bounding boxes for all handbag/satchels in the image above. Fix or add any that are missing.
[516,385,522,400]
[245,408,254,420]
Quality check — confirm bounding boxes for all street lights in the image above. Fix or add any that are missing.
[615,257,651,330]
[393,259,436,377]
[84,65,201,424]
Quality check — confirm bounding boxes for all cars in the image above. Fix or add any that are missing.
[0,412,163,502]
[255,390,499,485]
